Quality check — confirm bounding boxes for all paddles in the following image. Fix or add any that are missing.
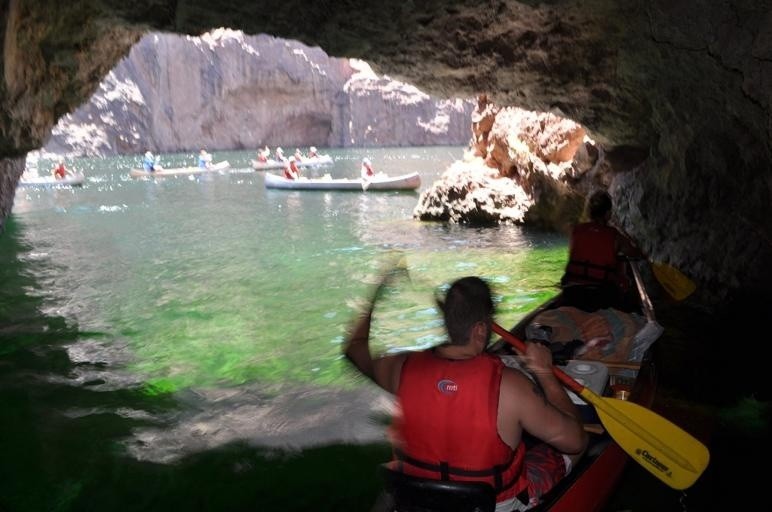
[609,219,696,301]
[490,321,710,491]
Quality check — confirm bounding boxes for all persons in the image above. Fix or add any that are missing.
[359,156,375,186]
[560,190,646,312]
[257,145,320,162]
[199,150,214,170]
[143,146,162,174]
[54,161,74,181]
[284,155,301,182]
[342,256,589,511]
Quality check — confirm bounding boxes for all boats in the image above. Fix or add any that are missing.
[249,154,336,172]
[358,283,674,512]
[16,172,84,188]
[129,159,230,178]
[261,167,421,192]
